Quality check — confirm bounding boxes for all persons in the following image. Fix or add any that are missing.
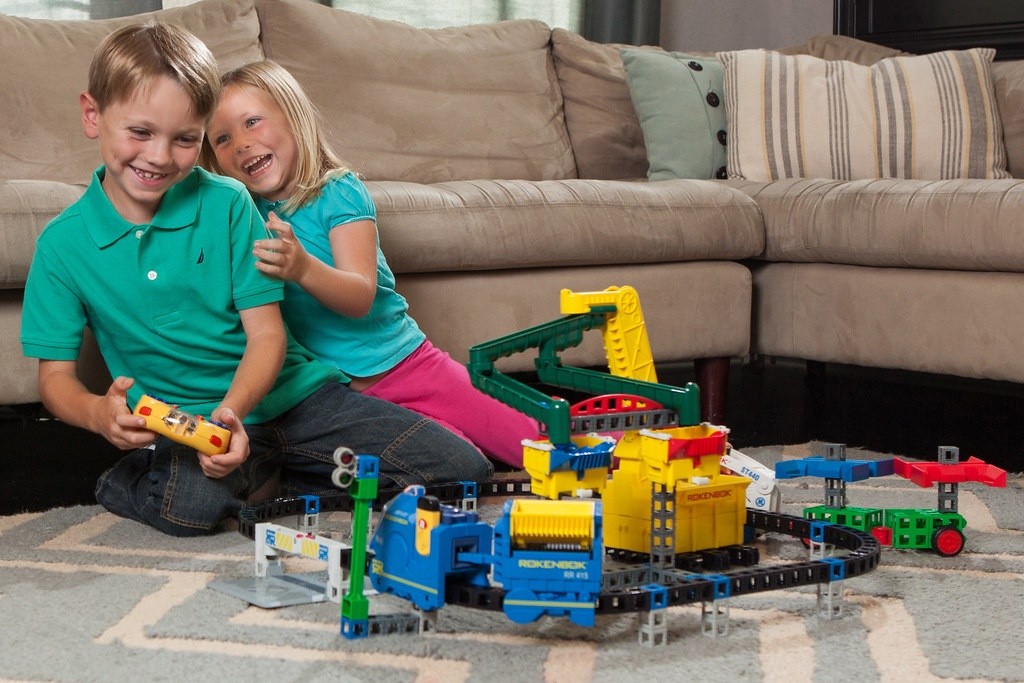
[20,23,492,537]
[200,61,622,471]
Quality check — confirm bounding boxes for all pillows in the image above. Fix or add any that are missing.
[715,47,1011,179]
[618,49,730,181]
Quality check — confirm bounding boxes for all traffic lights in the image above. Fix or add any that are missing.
[331,447,355,488]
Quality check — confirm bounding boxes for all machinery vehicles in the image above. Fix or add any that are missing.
[520,284,760,573]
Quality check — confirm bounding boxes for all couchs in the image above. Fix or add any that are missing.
[0,0,1024,518]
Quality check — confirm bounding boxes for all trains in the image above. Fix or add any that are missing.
[369,485,494,610]
